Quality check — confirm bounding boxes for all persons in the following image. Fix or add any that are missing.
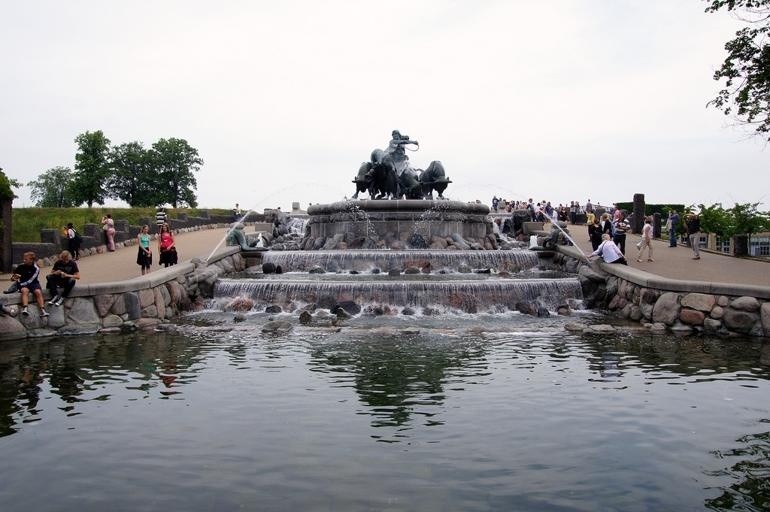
[10,205,178,317]
[233,204,240,216]
[492,195,701,266]
[387,130,423,187]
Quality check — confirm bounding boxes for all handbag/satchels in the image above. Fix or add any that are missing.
[103,219,109,231]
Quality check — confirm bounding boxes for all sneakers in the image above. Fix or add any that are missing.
[39,308,49,317]
[48,295,59,305]
[55,296,64,306]
[23,306,28,315]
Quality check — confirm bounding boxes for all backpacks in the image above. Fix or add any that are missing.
[71,228,83,243]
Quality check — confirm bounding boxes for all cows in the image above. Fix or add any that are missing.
[351,148,453,200]
